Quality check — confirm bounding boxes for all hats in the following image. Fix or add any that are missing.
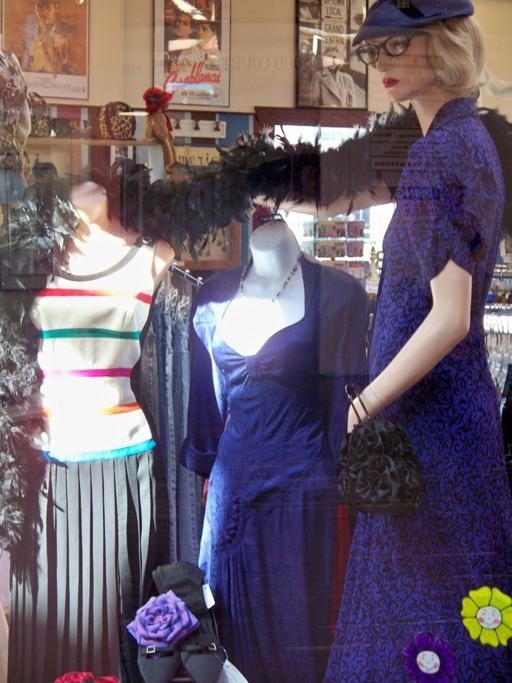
[351,0,476,46]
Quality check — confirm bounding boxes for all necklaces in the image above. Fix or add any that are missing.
[233,255,305,306]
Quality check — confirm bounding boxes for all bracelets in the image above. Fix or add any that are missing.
[351,380,374,423]
[340,384,367,431]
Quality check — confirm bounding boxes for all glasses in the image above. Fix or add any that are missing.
[354,29,434,65]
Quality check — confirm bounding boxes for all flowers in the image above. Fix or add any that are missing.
[126,589,200,652]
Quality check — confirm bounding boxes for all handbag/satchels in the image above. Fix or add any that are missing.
[331,379,426,517]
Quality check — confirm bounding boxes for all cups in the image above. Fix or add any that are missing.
[170,118,217,132]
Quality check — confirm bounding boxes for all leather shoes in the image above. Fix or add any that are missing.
[133,593,180,683]
[150,556,232,683]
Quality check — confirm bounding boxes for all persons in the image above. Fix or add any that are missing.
[313,1,510,680]
[180,205,370,681]
[3,174,179,683]
[22,0,72,75]
[308,46,357,108]
[163,1,221,83]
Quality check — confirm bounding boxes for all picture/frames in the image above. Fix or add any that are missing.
[0,0,90,100]
[294,0,369,111]
[152,0,231,109]
